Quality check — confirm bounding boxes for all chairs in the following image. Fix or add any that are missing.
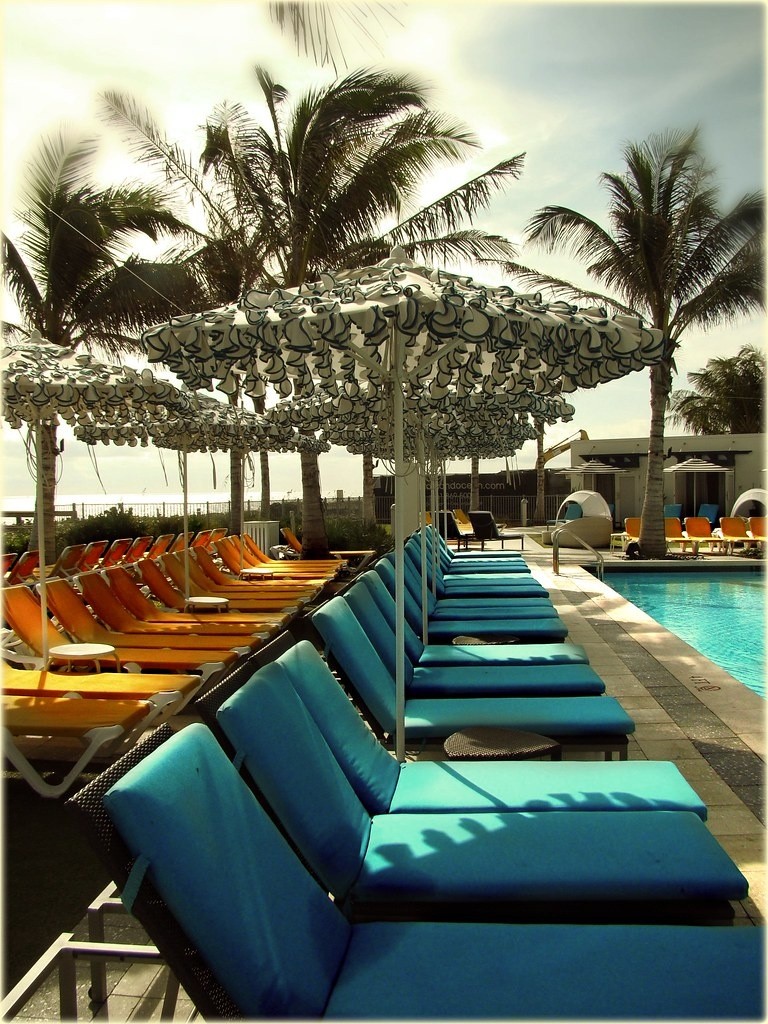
[0,503,766,1024]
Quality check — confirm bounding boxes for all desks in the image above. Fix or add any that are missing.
[329,550,377,574]
[42,640,120,673]
[239,568,275,580]
[610,533,630,555]
[183,594,232,614]
